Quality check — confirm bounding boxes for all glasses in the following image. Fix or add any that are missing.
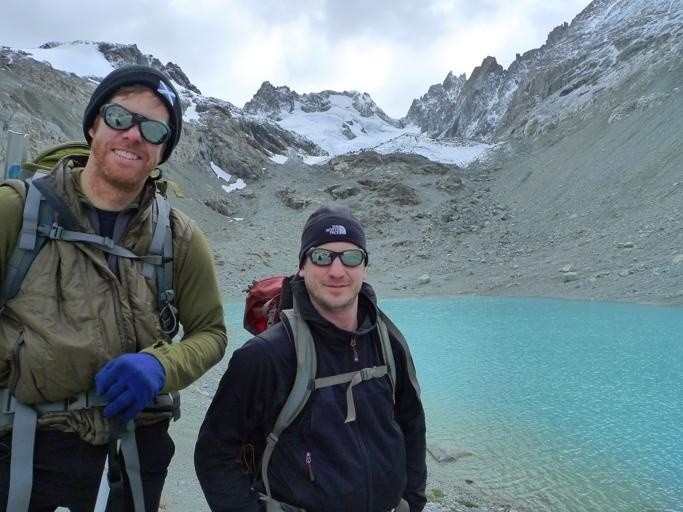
[308,249,368,267]
[98,105,168,150]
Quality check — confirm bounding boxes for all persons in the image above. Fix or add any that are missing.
[192,198,427,511]
[0,65,228,511]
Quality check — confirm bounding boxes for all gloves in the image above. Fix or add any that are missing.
[95,352,168,424]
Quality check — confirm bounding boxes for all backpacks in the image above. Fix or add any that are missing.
[243,275,397,512]
[0,139,179,430]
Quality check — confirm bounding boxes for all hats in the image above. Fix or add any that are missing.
[298,203,368,273]
[83,65,183,165]
